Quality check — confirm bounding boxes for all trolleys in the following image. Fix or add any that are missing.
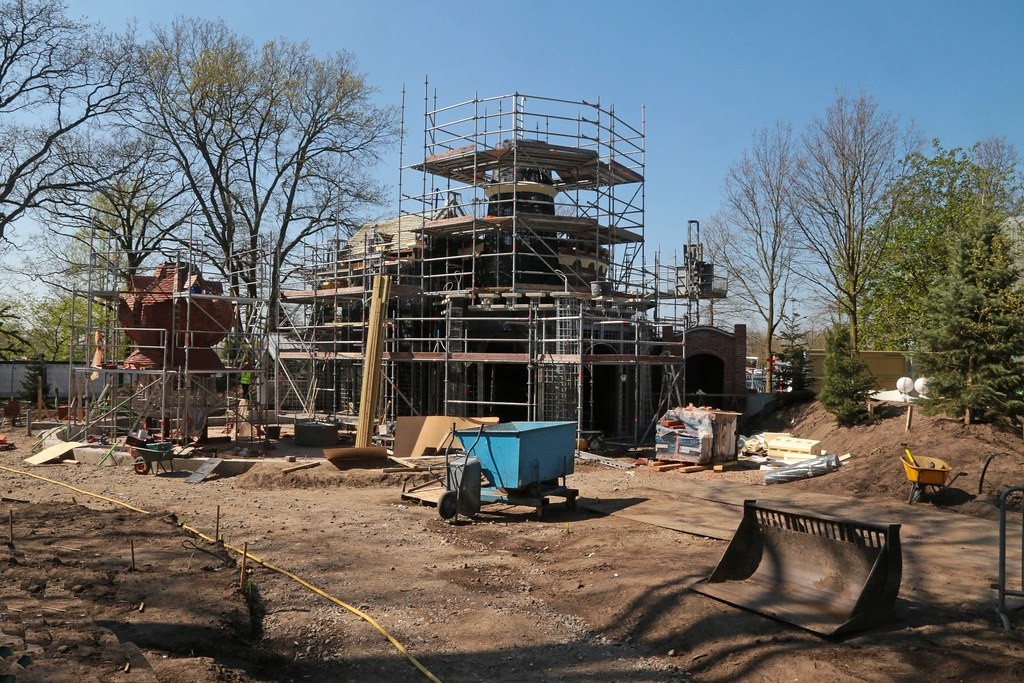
[899,449,968,505]
[128,444,175,473]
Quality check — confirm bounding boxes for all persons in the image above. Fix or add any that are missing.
[240,362,261,401]
